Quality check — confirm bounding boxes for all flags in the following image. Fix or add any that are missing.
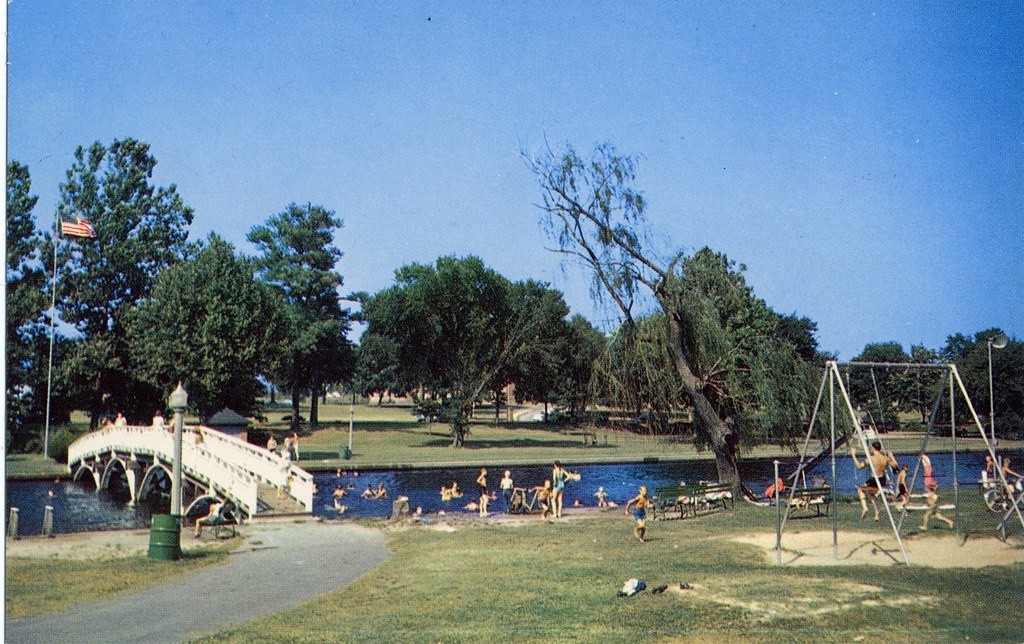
[60,213,98,238]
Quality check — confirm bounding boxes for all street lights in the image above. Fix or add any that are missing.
[168,379,188,558]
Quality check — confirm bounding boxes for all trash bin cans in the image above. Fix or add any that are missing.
[147,513,180,561]
[338,445,349,459]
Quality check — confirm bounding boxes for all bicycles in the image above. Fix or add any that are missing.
[984,476,1024,513]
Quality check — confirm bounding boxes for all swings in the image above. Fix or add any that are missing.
[846,368,896,493]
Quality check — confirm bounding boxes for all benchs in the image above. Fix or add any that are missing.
[786,487,832,519]
[199,505,236,538]
[653,482,734,521]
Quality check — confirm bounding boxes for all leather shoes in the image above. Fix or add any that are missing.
[679,583,693,589]
[652,585,667,593]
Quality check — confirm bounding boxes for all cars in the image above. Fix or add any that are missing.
[940,413,998,439]
[629,412,669,427]
[417,402,449,422]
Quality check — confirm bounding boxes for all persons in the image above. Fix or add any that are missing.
[986,457,993,485]
[625,485,653,543]
[153,410,164,429]
[910,481,954,531]
[193,498,230,538]
[277,452,293,499]
[267,436,277,453]
[167,411,185,433]
[898,464,912,509]
[1001,457,1024,500]
[920,452,933,485]
[193,424,204,456]
[333,470,424,517]
[115,411,127,427]
[501,460,620,518]
[439,467,492,516]
[850,441,898,522]
[101,417,113,428]
[284,433,299,461]
